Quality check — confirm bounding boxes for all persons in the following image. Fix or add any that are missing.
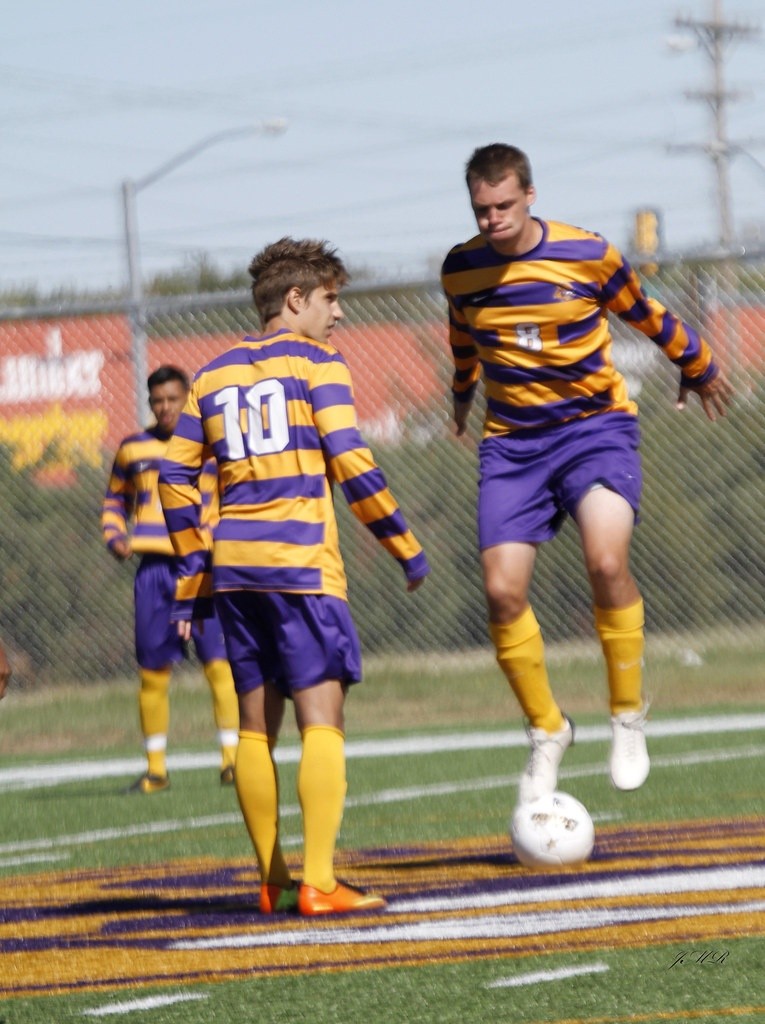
[441,143,737,807]
[101,364,241,793]
[156,236,426,913]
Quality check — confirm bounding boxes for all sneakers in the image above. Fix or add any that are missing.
[222,768,235,788]
[297,877,387,918]
[260,879,302,915]
[612,702,651,790]
[519,715,573,799]
[124,775,168,792]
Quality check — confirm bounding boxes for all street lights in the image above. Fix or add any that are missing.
[117,117,296,429]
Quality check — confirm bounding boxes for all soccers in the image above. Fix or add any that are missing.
[509,789,596,872]
